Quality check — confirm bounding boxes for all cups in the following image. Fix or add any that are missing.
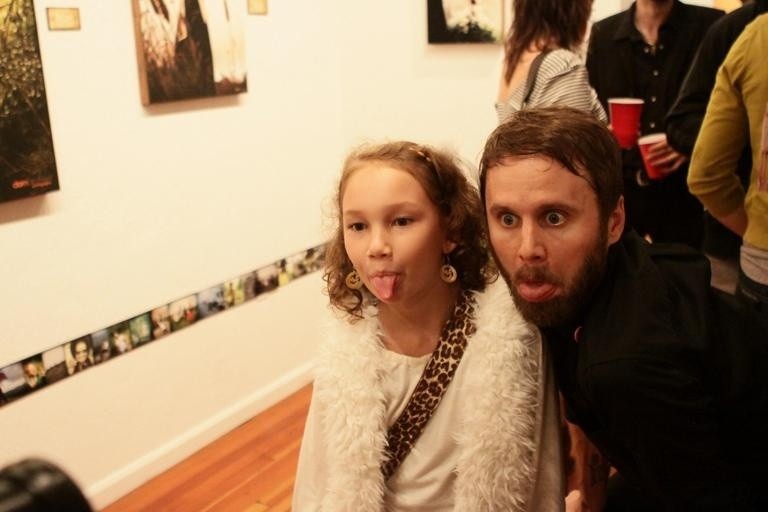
[635,132,671,180]
[607,97,645,151]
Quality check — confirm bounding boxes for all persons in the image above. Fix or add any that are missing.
[662,0,768,161]
[474,103,768,512]
[587,0,726,255]
[685,10,768,316]
[288,137,571,512]
[1,241,327,412]
[492,0,608,125]
